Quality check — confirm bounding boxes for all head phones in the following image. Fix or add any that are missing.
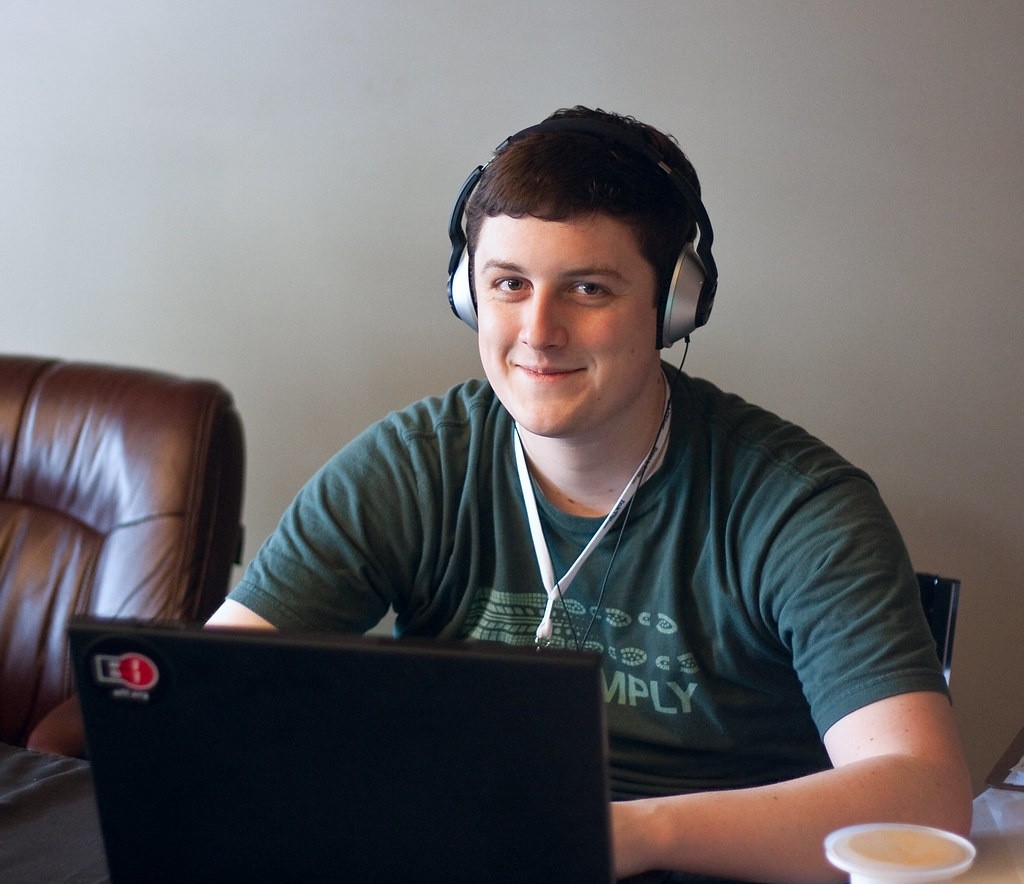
[446,116,718,350]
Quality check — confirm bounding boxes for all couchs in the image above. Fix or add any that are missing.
[0,354,245,762]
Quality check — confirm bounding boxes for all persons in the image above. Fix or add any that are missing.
[22,105,972,884]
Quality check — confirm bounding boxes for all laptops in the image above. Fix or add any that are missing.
[66,612,615,884]
